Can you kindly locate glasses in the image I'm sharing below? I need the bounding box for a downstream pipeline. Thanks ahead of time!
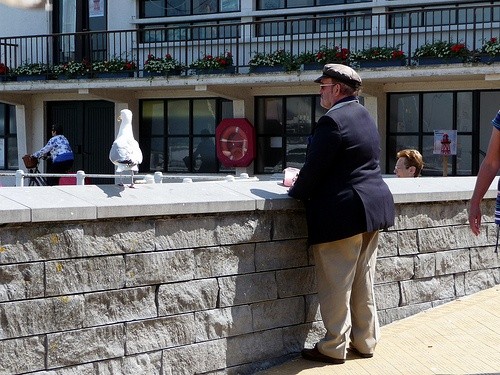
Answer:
[319,83,334,91]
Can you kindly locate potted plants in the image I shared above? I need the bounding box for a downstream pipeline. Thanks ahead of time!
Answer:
[59,168,91,184]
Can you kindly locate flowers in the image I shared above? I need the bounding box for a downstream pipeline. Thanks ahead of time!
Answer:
[0,38,500,76]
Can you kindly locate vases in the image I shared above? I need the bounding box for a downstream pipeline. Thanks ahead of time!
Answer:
[14,76,46,81]
[304,65,324,71]
[249,67,286,73]
[360,60,406,68]
[0,76,7,82]
[96,73,134,78]
[196,69,235,74]
[58,74,85,80]
[419,58,467,65]
[143,71,181,77]
[474,55,500,64]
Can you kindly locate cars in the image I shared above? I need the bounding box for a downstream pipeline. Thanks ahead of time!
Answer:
[272,153,306,173]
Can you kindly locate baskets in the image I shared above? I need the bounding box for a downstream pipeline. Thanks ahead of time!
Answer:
[23,156,37,168]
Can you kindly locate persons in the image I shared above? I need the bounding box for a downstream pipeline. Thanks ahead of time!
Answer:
[469,110,500,253]
[393,148,424,177]
[289,64,395,364]
[31,123,75,186]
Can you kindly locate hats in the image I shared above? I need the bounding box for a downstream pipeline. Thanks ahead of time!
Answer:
[314,63,362,90]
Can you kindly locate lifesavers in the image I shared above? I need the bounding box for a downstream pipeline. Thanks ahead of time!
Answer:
[220,126,248,161]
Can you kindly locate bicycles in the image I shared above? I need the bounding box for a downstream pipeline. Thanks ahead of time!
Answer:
[22,153,91,186]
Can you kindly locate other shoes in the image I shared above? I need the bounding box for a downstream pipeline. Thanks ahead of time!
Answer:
[349,342,373,358]
[300,343,344,363]
